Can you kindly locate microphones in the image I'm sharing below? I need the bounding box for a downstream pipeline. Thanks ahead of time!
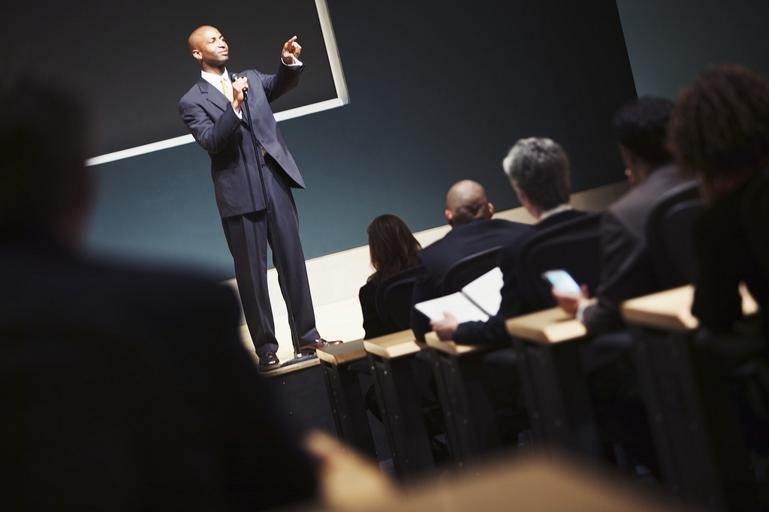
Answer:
[231,73,248,95]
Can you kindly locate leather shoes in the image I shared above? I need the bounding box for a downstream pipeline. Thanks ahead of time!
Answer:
[293,337,343,358]
[259,352,280,371]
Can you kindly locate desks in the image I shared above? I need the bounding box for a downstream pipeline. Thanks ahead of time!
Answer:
[317,284,760,483]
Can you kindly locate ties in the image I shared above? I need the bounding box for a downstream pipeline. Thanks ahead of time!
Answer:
[219,77,232,100]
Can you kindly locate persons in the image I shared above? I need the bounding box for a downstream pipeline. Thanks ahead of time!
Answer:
[176,25,344,373]
[2,71,322,511]
